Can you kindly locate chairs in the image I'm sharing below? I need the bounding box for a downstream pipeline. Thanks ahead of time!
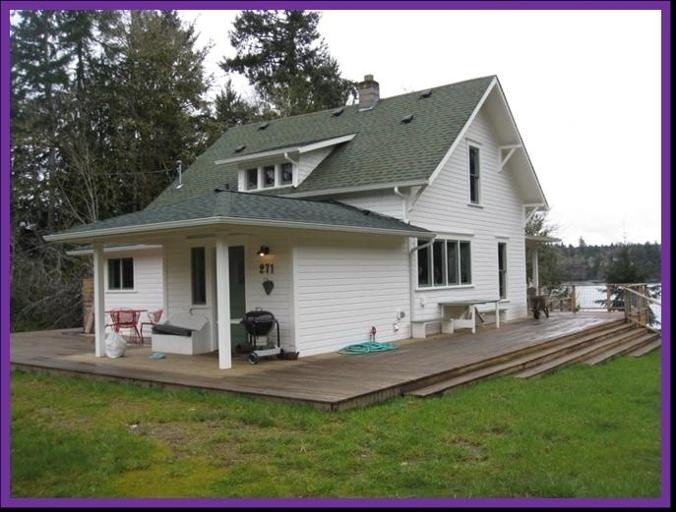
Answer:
[103,306,162,345]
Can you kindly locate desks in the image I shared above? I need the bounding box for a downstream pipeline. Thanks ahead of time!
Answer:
[436,296,500,334]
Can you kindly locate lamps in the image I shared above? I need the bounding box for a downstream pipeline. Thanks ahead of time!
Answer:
[256,245,269,256]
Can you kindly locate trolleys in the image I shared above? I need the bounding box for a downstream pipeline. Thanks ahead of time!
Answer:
[531,294,550,319]
[236,309,286,364]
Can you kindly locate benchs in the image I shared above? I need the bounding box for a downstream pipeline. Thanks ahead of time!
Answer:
[478,306,508,326]
[410,317,454,339]
[150,311,212,355]
